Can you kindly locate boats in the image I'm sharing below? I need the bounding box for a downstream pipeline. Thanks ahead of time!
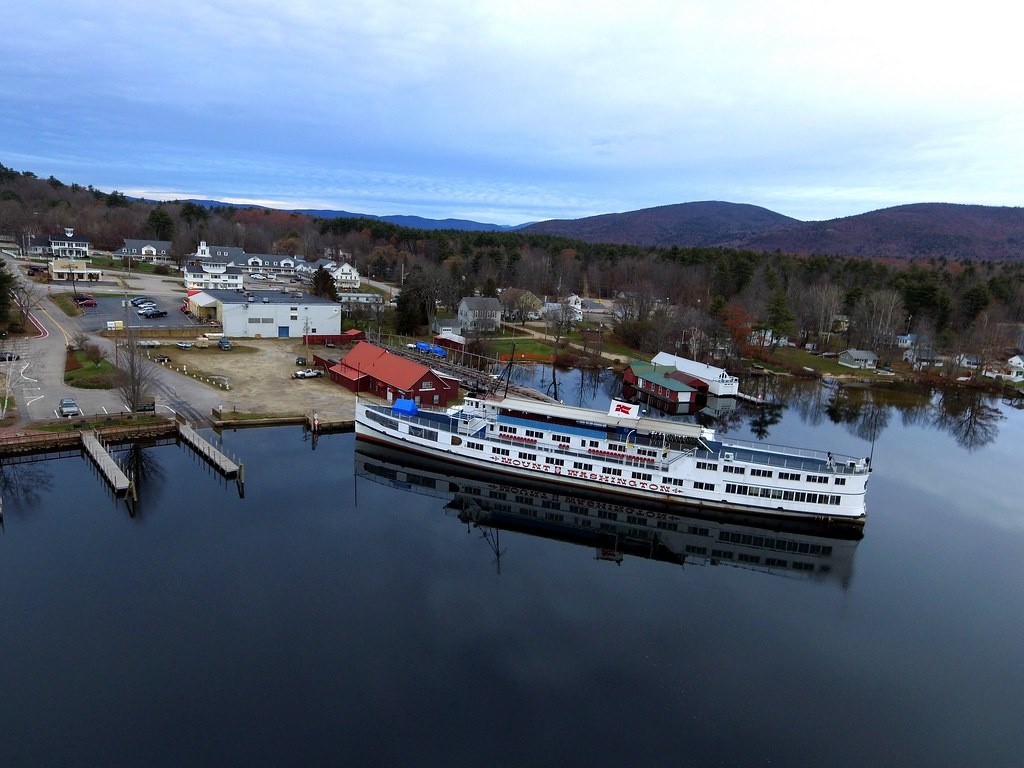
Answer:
[175,342,192,351]
[351,446,867,582]
[355,337,878,524]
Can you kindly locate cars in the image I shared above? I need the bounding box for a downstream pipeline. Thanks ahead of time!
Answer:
[71,292,98,307]
[59,398,79,417]
[295,357,307,367]
[0,351,20,362]
[130,296,168,319]
[242,291,269,304]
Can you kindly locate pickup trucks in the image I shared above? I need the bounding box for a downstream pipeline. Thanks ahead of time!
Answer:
[294,368,325,380]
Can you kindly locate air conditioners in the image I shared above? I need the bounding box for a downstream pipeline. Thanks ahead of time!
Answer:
[724,452,734,460]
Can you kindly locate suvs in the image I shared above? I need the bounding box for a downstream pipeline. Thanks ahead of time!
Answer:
[218,339,231,351]
